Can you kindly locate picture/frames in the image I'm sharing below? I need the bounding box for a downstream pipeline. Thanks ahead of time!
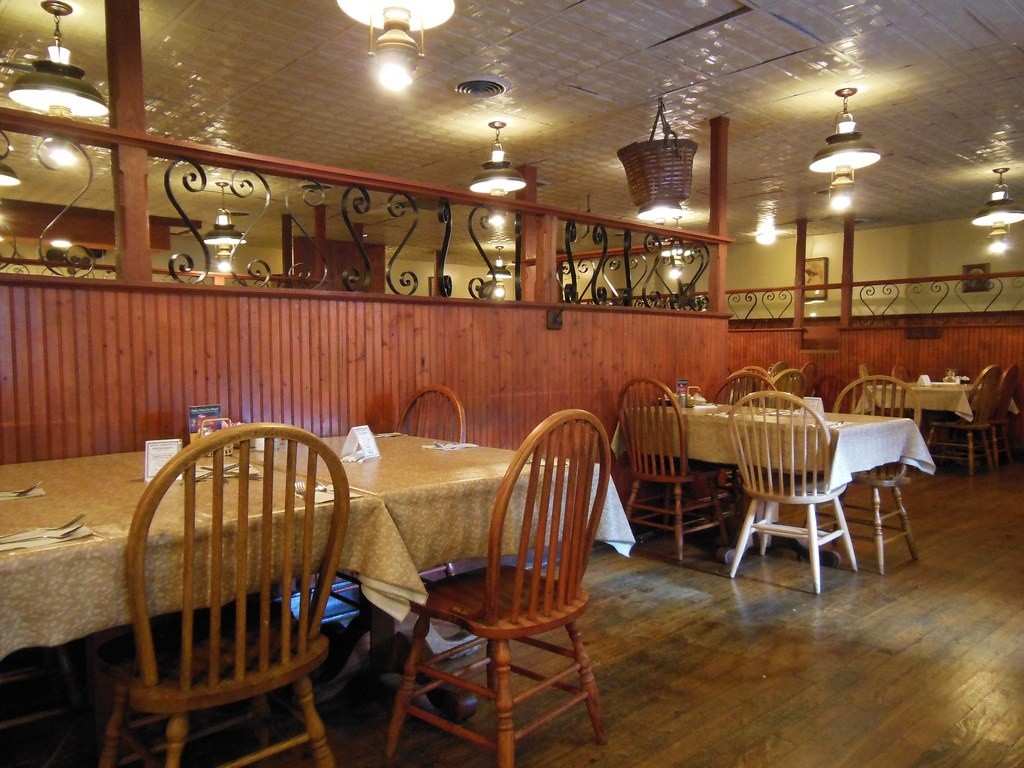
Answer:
[962,263,991,292]
[804,257,828,300]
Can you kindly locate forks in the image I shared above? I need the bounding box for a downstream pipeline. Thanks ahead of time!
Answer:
[12,481,40,493]
[0,513,85,539]
[294,482,306,495]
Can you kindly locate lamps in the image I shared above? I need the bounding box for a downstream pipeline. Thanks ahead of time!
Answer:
[618,96,700,223]
[486,246,512,296]
[0,143,22,188]
[204,180,247,272]
[809,89,881,213]
[9,0,109,171]
[971,167,1024,254]
[660,217,693,278]
[753,227,778,246]
[335,0,455,91]
[470,121,528,225]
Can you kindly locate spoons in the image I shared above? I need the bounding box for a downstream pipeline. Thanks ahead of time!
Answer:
[828,421,844,427]
[434,440,447,448]
[0,523,84,545]
[0,486,37,497]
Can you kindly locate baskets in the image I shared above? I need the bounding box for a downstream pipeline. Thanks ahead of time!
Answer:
[617,139,698,221]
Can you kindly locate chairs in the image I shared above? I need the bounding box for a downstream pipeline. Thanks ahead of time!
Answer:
[616,375,730,563]
[858,362,1019,475]
[727,390,857,594]
[713,373,779,550]
[729,359,818,397]
[327,385,466,636]
[91,422,349,768]
[385,409,610,768]
[796,376,919,576]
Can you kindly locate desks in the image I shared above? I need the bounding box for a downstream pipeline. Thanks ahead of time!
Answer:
[611,400,936,562]
[866,380,1019,422]
[0,432,636,728]
